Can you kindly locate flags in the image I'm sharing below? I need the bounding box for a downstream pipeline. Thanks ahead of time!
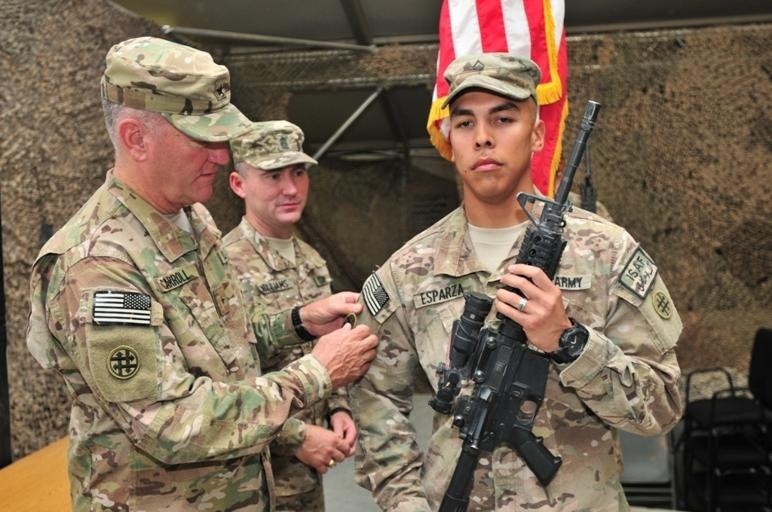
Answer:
[425,1,570,203]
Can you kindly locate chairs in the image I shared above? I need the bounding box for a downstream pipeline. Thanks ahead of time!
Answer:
[677,326,771,511]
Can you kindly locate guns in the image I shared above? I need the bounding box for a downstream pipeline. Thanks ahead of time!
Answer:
[428,100,602,512]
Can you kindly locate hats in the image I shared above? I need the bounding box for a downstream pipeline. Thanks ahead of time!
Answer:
[99,36,254,146]
[441,53,541,110]
[227,119,318,172]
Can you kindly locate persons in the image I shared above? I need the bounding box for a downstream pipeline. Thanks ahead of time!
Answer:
[349,52,685,512]
[219,119,358,511]
[27,35,381,512]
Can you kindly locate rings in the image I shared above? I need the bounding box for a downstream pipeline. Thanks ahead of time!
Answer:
[518,298,528,312]
[329,458,337,468]
